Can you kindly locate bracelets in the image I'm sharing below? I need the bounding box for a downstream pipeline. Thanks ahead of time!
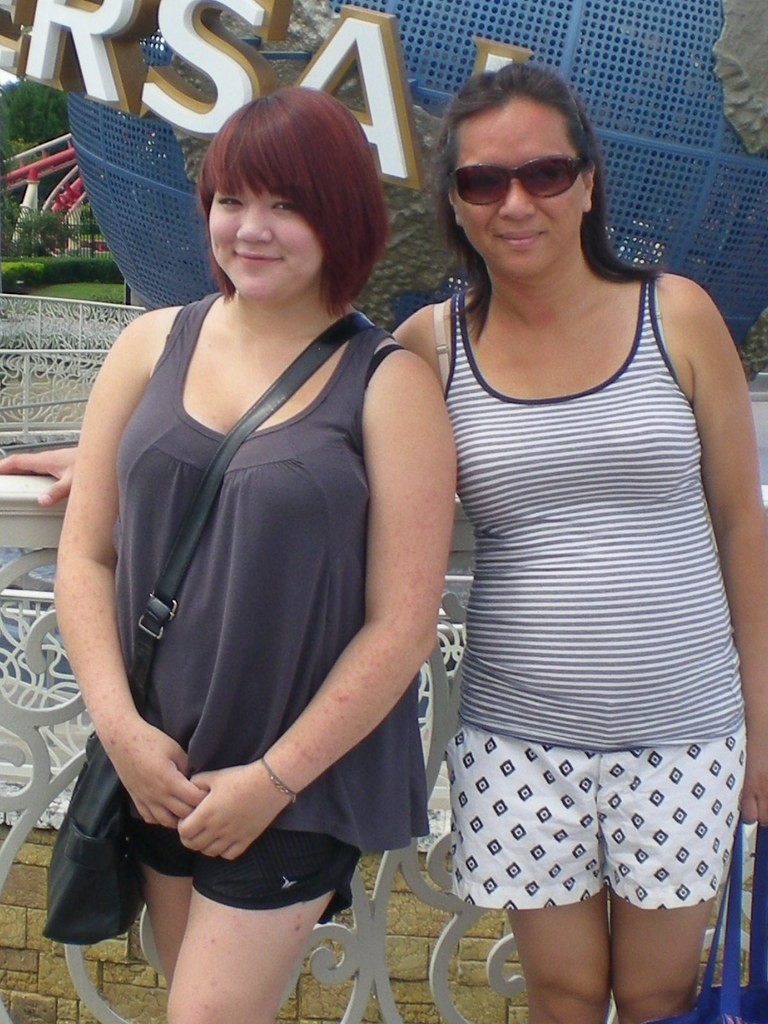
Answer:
[261,757,297,803]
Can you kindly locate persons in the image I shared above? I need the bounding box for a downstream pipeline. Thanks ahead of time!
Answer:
[0,64,767,1023]
[52,85,459,1023]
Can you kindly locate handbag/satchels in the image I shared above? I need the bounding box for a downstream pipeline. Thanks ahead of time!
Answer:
[643,811,768,1024]
[41,730,149,947]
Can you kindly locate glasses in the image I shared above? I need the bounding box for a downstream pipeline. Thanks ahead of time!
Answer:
[448,154,588,207]
